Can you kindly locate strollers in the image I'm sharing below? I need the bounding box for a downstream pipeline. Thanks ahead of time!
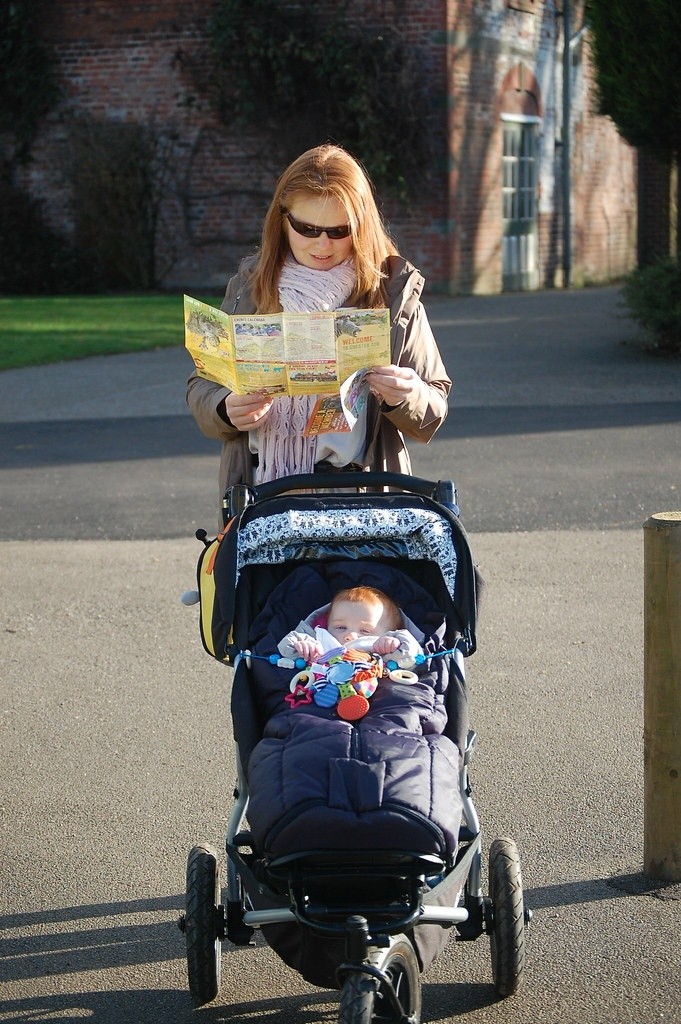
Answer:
[177,469,532,1024]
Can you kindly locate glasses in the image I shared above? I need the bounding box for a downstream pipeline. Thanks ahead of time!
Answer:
[283,208,351,239]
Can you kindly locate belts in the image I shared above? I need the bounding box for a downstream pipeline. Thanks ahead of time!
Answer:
[250,453,363,473]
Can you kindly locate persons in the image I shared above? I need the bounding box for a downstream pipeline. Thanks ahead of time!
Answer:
[186,144,453,532]
[247,586,465,895]
